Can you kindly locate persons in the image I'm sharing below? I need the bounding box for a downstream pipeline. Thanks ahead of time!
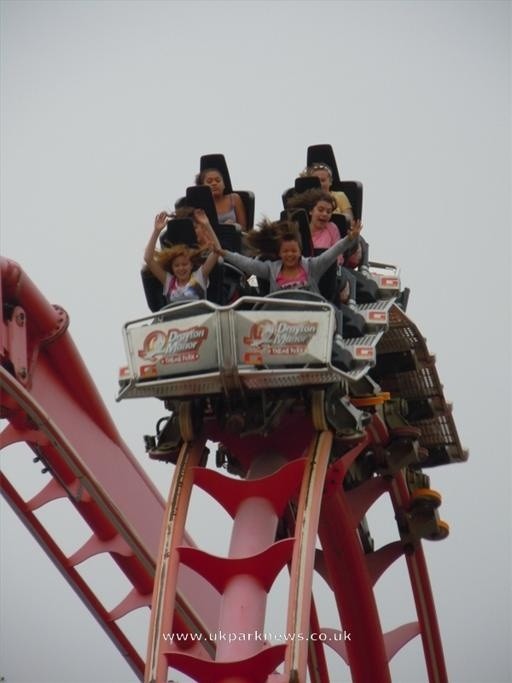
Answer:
[296,163,357,224]
[141,206,223,307]
[282,190,352,304]
[209,213,364,304]
[194,167,249,233]
[165,198,224,263]
